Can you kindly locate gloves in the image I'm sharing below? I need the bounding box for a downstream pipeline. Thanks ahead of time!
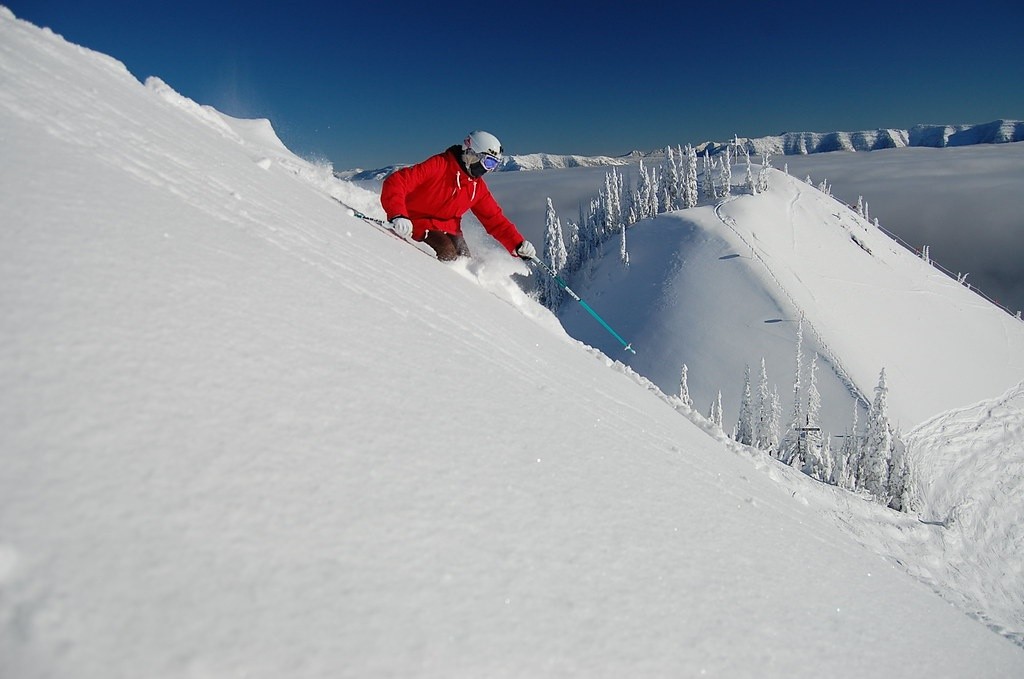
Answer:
[518,240,536,258]
[392,218,413,238]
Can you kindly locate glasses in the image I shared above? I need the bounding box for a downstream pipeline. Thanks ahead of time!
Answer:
[477,153,500,171]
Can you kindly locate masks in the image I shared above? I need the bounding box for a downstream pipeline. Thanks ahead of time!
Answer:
[469,161,488,177]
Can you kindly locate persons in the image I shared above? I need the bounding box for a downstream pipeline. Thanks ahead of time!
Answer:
[379,128,537,263]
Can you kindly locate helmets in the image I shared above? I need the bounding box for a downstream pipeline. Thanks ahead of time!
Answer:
[462,130,504,159]
